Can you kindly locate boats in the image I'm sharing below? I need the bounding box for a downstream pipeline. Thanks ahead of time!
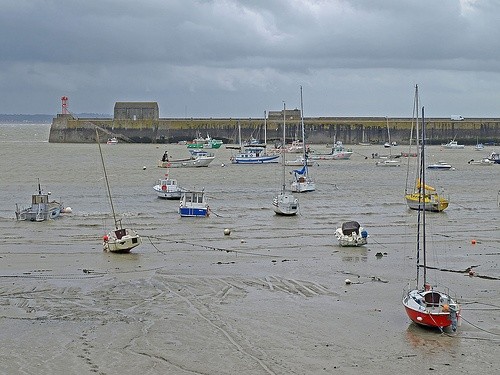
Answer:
[426,161,451,170]
[158,100,420,168]
[333,220,370,247]
[474,136,486,151]
[177,186,210,218]
[467,151,500,165]
[152,169,186,200]
[14,178,72,223]
[441,132,465,149]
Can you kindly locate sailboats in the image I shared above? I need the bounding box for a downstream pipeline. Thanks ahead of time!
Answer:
[404,83,449,211]
[272,100,299,216]
[402,106,462,333]
[288,84,316,194]
[95,128,143,255]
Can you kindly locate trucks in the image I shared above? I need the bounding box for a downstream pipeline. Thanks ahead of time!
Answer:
[450,115,464,121]
